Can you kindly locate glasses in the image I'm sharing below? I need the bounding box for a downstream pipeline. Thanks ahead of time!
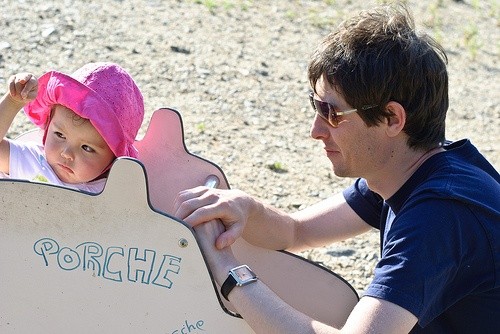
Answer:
[309,93,379,127]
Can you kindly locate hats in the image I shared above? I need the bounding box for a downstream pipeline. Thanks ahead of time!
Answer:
[24,63,145,158]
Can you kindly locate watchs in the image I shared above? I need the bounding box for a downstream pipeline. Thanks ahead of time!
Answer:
[220,264,260,302]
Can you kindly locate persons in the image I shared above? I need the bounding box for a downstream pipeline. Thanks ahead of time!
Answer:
[168,0,500,334]
[0,66,141,198]
